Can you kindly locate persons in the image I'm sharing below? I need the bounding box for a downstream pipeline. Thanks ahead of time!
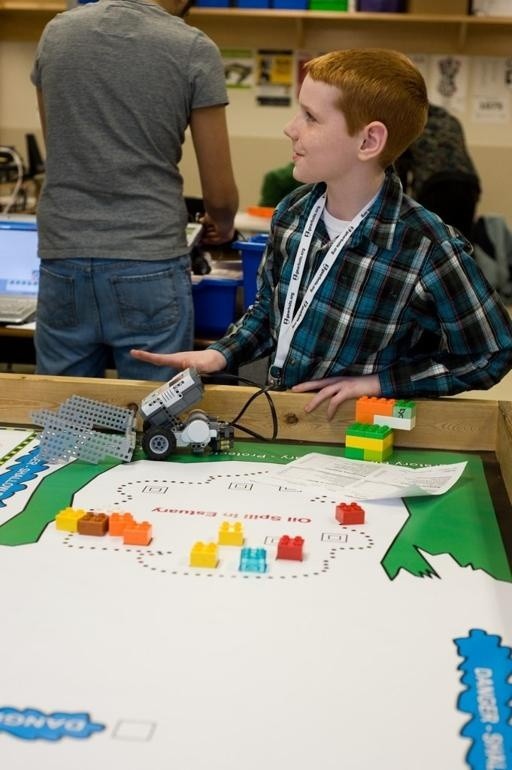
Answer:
[30,1,240,383]
[130,47,511,422]
[395,106,511,307]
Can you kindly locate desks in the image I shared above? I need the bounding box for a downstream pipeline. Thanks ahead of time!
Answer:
[0,373,512,770]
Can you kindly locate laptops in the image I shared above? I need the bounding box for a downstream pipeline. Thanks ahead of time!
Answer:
[0,213,42,325]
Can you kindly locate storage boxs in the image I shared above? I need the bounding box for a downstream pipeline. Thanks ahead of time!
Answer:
[233,234,269,313]
[196,279,243,338]
